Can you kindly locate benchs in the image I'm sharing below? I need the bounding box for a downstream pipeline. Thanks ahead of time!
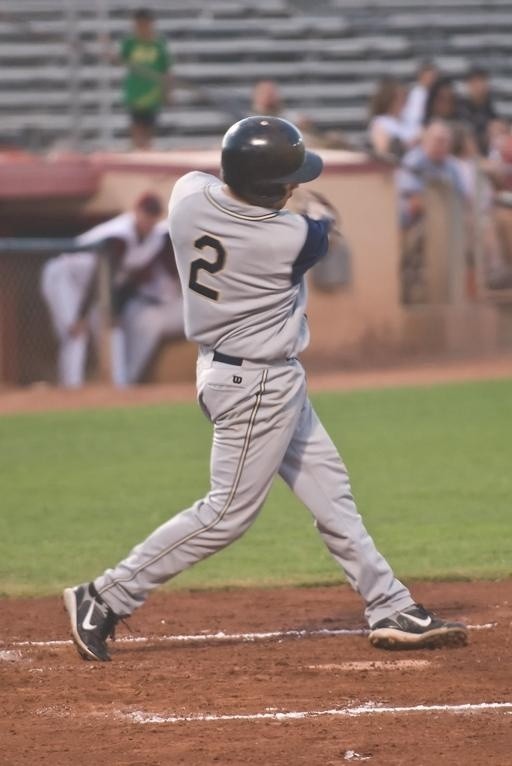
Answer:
[1,1,512,150]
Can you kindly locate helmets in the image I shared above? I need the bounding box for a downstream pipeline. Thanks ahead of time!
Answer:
[220,115,324,184]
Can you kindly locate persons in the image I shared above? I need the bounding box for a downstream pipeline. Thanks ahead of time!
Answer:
[115,237,186,386]
[57,111,471,667]
[242,80,325,150]
[366,55,512,291]
[107,8,178,152]
[40,192,170,385]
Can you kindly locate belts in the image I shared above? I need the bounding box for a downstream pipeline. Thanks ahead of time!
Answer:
[212,351,243,366]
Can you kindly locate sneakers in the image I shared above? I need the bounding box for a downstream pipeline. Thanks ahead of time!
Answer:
[368,602,469,650]
[63,582,115,662]
